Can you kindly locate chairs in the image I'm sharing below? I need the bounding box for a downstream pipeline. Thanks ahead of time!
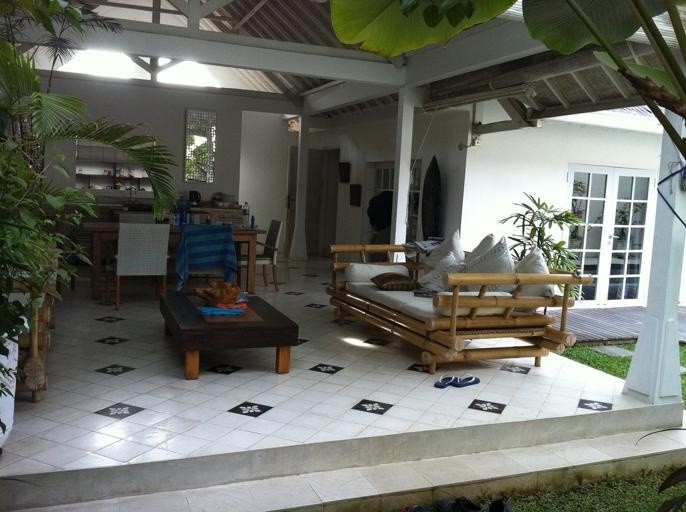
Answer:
[327,240,594,373]
[113,209,245,309]
[235,220,284,292]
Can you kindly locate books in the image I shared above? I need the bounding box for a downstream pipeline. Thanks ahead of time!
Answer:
[414,240,441,254]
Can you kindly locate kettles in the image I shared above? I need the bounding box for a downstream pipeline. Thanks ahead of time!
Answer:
[189,190,202,205]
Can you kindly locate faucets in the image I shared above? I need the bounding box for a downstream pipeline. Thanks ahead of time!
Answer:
[128,185,136,202]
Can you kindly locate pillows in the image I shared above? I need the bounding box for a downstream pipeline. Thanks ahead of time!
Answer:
[416,228,552,299]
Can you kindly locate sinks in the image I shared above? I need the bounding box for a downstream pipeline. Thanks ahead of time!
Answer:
[122,203,152,211]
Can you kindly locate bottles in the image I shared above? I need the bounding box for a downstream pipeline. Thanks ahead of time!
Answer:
[241,201,250,227]
[252,215,256,229]
